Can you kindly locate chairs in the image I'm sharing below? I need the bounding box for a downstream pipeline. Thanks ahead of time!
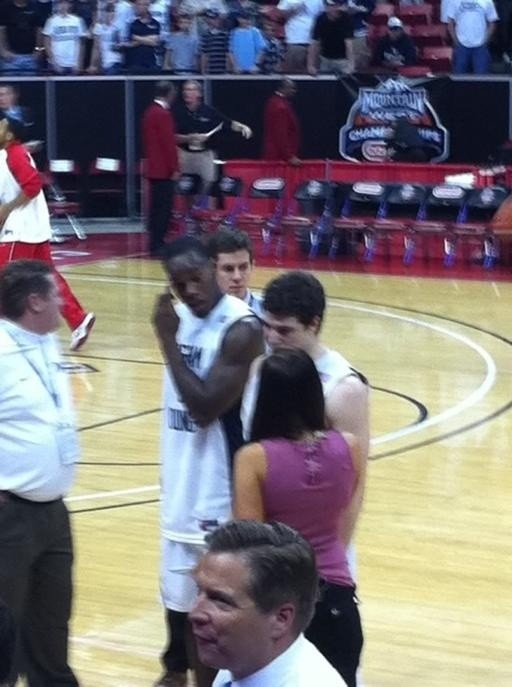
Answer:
[252,0,455,79]
[38,171,87,242]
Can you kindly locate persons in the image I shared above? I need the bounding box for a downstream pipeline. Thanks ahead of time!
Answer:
[239,271,370,588]
[0,259,80,687]
[144,80,180,259]
[234,348,367,687]
[173,78,252,153]
[0,0,512,78]
[385,116,429,165]
[187,522,347,687]
[260,79,302,165]
[1,83,96,351]
[487,138,511,166]
[154,237,266,687]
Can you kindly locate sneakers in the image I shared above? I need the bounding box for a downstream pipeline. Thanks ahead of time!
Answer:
[152,670,188,687]
[68,311,96,351]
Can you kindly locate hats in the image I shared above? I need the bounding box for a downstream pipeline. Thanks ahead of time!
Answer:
[387,17,405,30]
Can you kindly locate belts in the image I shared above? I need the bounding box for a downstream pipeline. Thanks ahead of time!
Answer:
[1,490,25,503]
